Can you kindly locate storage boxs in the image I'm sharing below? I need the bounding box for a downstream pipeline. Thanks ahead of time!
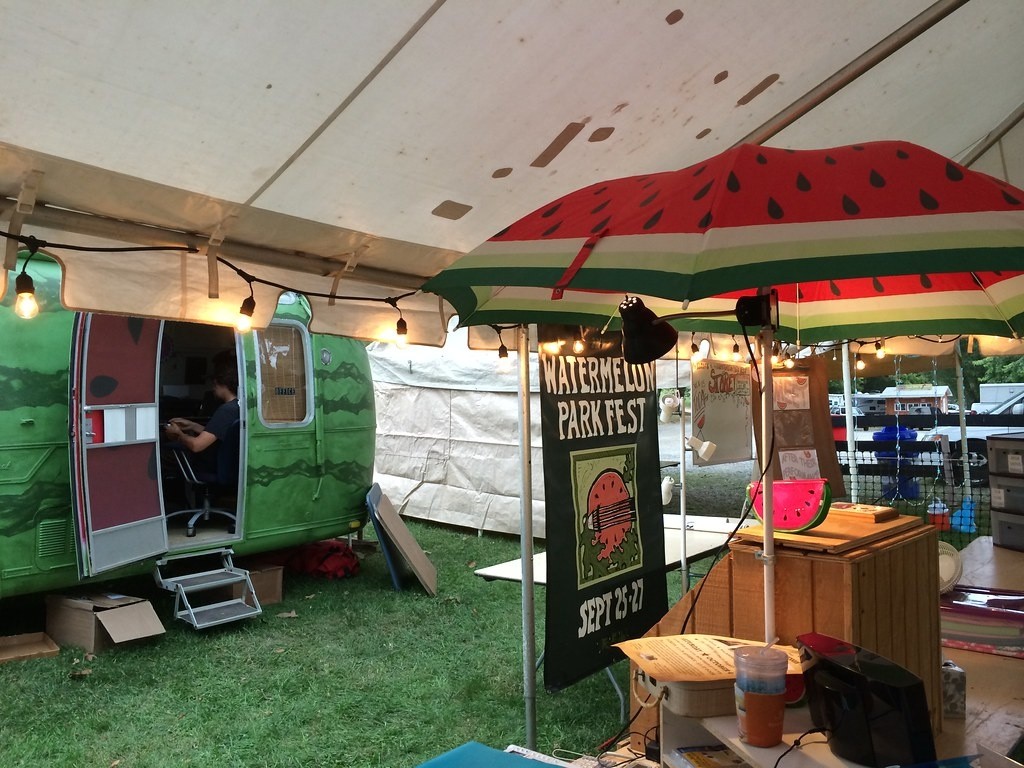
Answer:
[0,632,60,664]
[45,591,166,654]
[986,432,1024,552]
[222,562,284,605]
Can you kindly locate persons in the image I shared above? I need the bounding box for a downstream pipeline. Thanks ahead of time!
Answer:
[165,375,239,525]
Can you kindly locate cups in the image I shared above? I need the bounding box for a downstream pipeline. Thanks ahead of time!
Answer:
[734,646,789,694]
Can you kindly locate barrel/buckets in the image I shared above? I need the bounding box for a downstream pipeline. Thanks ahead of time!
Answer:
[926,497,950,531]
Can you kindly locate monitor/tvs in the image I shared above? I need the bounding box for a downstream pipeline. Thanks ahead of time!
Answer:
[795,631,939,768]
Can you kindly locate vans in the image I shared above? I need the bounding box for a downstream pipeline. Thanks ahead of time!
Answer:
[0,249,378,632]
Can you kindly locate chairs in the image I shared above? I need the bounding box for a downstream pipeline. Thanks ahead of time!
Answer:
[164,441,236,538]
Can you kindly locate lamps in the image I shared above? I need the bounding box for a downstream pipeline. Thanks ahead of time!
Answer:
[661,477,675,506]
[618,294,768,365]
[659,396,679,424]
[685,436,717,461]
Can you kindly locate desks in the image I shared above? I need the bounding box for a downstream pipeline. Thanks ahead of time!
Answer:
[474,514,761,725]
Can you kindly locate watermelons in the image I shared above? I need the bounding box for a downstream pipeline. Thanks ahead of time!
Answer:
[746,479,831,534]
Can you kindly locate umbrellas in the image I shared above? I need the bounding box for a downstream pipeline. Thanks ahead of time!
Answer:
[782,332,1024,379]
[414,141,1023,637]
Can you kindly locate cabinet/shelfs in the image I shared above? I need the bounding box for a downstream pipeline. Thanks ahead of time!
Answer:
[660,701,900,768]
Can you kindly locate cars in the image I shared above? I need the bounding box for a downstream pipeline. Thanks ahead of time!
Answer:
[916,391,1024,488]
[831,406,869,431]
[908,406,943,430]
[948,404,977,415]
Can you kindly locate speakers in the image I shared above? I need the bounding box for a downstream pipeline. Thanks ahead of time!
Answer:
[736,295,771,326]
